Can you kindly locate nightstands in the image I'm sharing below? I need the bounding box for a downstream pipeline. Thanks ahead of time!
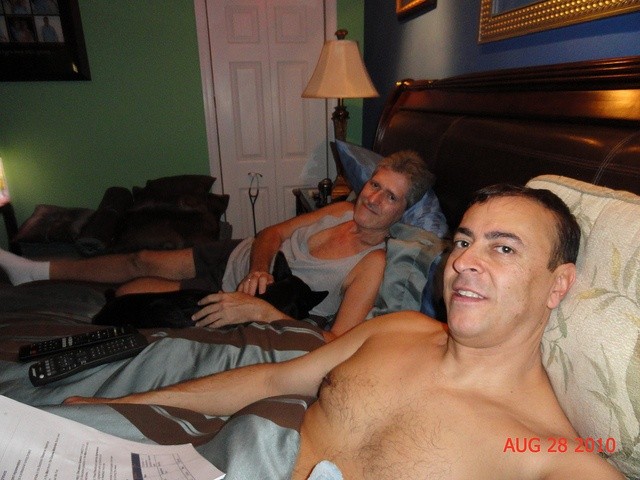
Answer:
[292,187,348,217]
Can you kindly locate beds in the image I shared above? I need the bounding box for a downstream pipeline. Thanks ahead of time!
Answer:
[1,58,640,480]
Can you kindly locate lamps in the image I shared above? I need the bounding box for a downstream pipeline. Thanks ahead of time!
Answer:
[301,30,379,198]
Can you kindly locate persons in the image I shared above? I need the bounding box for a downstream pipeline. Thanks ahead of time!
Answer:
[0,150,427,344]
[62,183,628,480]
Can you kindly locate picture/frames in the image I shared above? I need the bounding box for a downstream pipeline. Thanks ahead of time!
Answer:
[477,0,640,45]
[1,0,95,82]
[394,0,425,15]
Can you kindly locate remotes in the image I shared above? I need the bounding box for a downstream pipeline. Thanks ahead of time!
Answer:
[30,333,149,385]
[19,324,139,363]
